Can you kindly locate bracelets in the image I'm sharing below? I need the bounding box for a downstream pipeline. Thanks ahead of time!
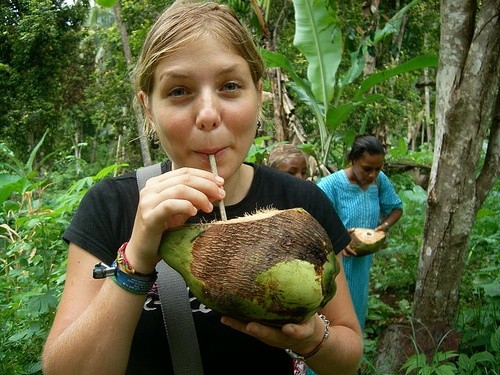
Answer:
[285,314,330,360]
[384,221,390,229]
[93,241,158,295]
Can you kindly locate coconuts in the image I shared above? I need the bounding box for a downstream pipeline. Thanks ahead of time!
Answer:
[158,206,341,328]
[348,228,387,256]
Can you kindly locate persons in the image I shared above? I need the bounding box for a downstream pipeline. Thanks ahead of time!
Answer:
[267,143,308,374]
[40,1,364,375]
[303,134,403,374]
